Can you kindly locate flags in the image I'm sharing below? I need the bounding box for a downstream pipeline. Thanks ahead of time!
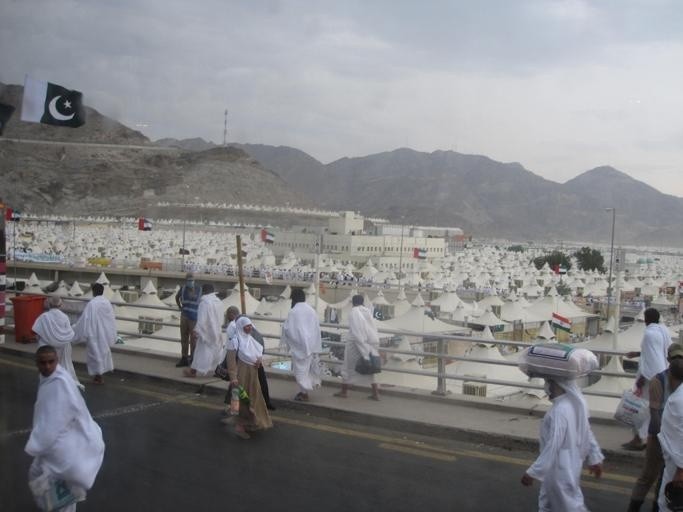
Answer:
[413,247,426,259]
[138,218,152,231]
[20,73,86,129]
[260,229,274,244]
[4,207,21,222]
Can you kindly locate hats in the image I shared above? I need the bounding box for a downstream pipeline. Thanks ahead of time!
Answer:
[668,343,683,358]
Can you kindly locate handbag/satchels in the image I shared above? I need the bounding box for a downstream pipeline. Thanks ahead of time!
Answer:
[28,464,87,512]
[355,352,382,374]
[614,388,650,429]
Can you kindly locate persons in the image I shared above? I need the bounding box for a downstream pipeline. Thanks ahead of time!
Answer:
[174,271,382,441]
[70,283,117,385]
[24,344,104,512]
[32,295,81,391]
[520,308,683,512]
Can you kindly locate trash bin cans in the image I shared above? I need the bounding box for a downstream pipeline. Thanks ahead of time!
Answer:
[9,295,47,344]
